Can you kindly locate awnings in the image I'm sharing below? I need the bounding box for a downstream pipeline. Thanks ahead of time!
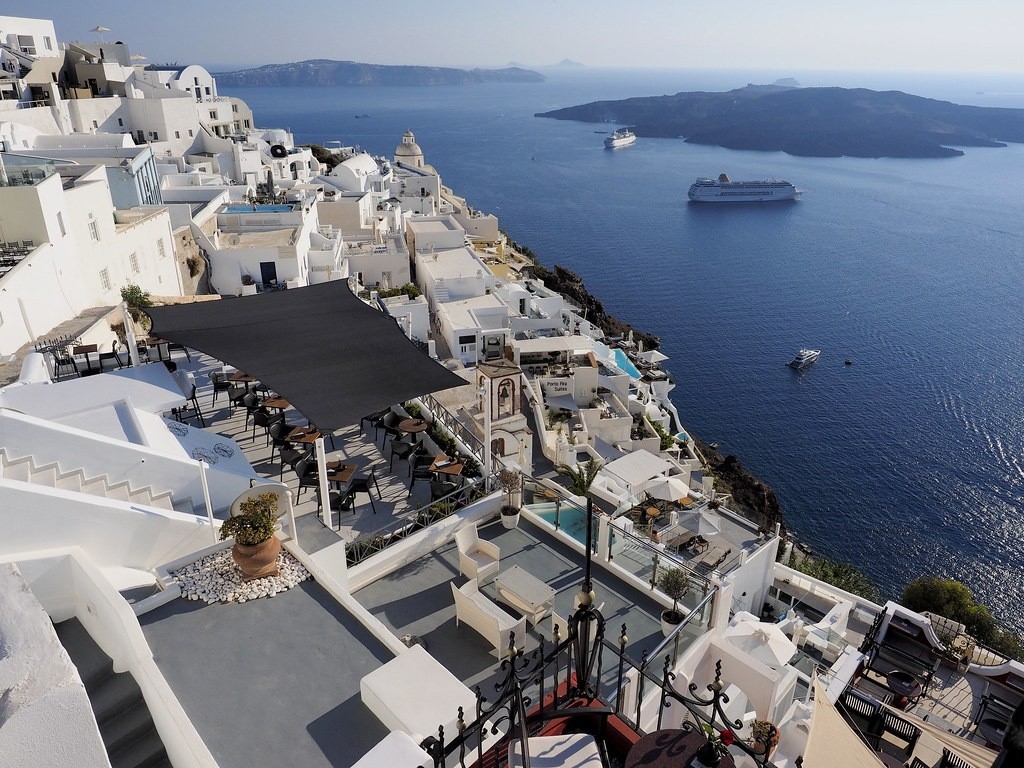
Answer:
[594,434,628,464]
[138,276,472,440]
[544,392,580,412]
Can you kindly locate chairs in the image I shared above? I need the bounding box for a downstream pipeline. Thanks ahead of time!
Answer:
[974,672,1024,725]
[98,340,124,373]
[452,523,502,583]
[407,453,439,499]
[175,384,206,429]
[167,343,193,363]
[294,459,323,505]
[429,476,465,503]
[348,464,382,514]
[903,754,930,768]
[314,481,355,530]
[838,690,881,734]
[853,727,880,751]
[52,353,80,380]
[880,711,922,757]
[550,603,605,650]
[505,681,613,768]
[126,341,151,367]
[208,370,235,408]
[228,368,469,482]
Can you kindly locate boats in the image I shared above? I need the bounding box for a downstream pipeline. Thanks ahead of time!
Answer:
[603,128,636,148]
[789,348,820,369]
[687,173,796,202]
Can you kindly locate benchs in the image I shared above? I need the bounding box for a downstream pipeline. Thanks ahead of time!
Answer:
[849,674,895,709]
[449,577,528,662]
[863,641,942,704]
[699,546,731,571]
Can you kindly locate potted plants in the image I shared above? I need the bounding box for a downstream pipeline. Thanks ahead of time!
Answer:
[218,491,283,582]
[495,470,522,530]
[658,566,689,640]
[749,718,781,756]
[763,602,773,619]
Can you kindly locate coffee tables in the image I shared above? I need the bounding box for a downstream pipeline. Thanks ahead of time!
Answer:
[493,565,558,628]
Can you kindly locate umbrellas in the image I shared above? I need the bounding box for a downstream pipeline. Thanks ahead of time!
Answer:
[644,476,690,518]
[130,54,146,64]
[638,349,670,371]
[727,619,797,668]
[89,25,112,43]
[677,509,720,554]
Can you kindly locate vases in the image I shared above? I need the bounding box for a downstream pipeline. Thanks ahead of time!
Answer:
[697,742,722,766]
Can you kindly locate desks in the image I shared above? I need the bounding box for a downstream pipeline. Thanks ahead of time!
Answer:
[316,461,358,489]
[262,392,290,413]
[430,454,468,481]
[284,427,320,450]
[398,419,428,443]
[229,370,255,393]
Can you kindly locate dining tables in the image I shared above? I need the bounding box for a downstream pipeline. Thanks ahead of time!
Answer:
[72,344,99,377]
[146,338,177,373]
[624,729,735,768]
[884,672,924,711]
[695,541,709,553]
[979,718,1007,752]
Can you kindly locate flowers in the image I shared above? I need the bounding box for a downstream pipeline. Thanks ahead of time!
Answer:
[702,723,733,746]
